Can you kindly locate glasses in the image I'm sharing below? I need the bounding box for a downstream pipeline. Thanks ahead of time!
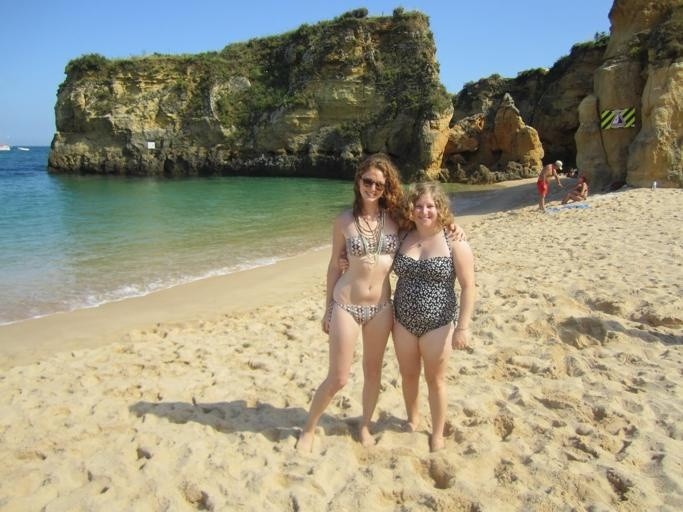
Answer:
[360,177,386,191]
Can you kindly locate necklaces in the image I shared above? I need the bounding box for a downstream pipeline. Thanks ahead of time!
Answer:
[416,232,429,246]
[353,208,385,271]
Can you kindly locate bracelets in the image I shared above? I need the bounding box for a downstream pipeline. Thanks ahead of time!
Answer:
[456,325,469,331]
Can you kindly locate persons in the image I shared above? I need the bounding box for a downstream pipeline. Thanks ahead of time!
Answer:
[562,176,588,204]
[339,181,474,453]
[537,160,563,210]
[295,154,466,453]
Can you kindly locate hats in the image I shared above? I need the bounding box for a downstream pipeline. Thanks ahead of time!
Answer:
[555,160,563,170]
